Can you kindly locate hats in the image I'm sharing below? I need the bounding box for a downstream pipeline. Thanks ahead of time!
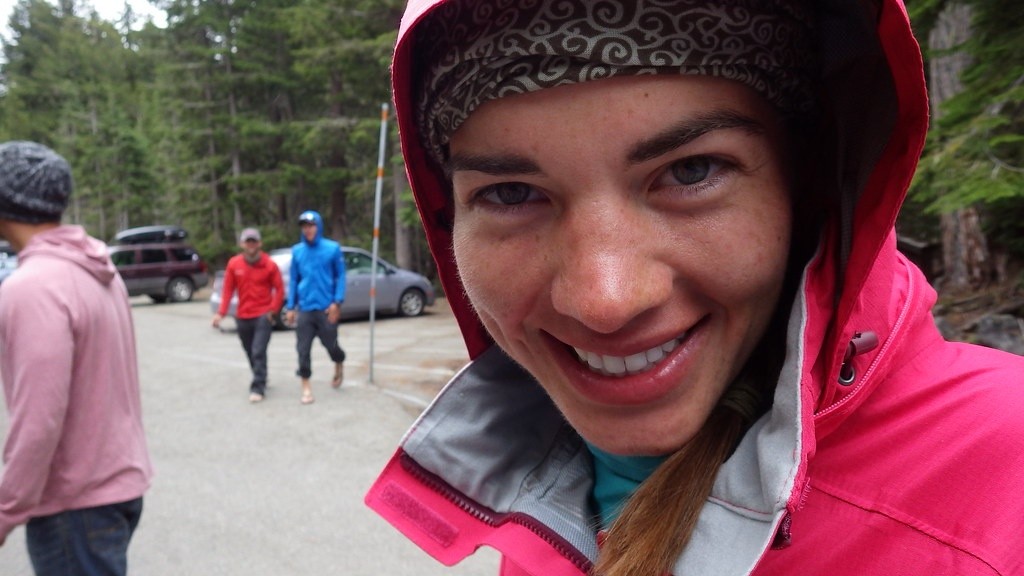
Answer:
[0,140,73,224]
[297,210,324,223]
[240,227,263,243]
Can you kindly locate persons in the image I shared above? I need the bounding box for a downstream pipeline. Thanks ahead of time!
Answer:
[210,229,284,403]
[0,142,155,575]
[365,1,1024,576]
[285,210,347,404]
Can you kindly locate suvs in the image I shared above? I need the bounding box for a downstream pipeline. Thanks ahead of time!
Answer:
[108,226,209,305]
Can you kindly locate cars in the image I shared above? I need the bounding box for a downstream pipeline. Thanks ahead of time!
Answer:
[210,240,434,327]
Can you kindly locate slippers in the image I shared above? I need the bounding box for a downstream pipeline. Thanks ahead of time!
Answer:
[300,389,316,406]
[249,392,265,405]
[331,368,343,388]
